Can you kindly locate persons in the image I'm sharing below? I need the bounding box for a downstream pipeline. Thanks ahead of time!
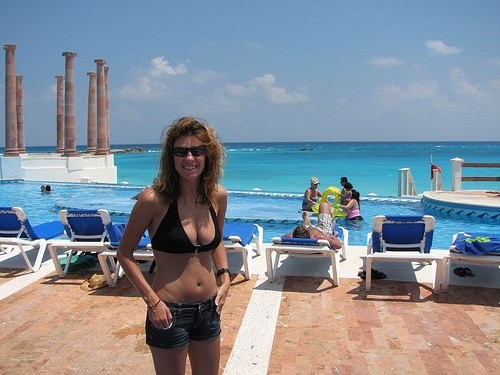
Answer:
[40,184,51,192]
[115,118,231,375]
[280,175,364,250]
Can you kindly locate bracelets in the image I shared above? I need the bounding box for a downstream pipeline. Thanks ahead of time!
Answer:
[217,268,231,279]
[147,299,161,310]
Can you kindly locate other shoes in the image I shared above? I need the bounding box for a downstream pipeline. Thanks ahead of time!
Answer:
[454,267,475,277]
[358,268,386,279]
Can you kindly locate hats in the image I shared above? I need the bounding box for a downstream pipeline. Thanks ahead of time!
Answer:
[311,177,320,184]
[81,272,111,291]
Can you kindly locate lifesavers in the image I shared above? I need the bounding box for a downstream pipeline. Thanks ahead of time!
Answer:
[311,204,346,217]
[321,187,341,207]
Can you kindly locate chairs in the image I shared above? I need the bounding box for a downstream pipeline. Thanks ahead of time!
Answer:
[217,222,263,281]
[0,204,64,273]
[360,215,500,292]
[265,226,349,286]
[47,209,155,288]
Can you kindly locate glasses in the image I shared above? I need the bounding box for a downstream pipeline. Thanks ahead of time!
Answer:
[172,147,205,157]
